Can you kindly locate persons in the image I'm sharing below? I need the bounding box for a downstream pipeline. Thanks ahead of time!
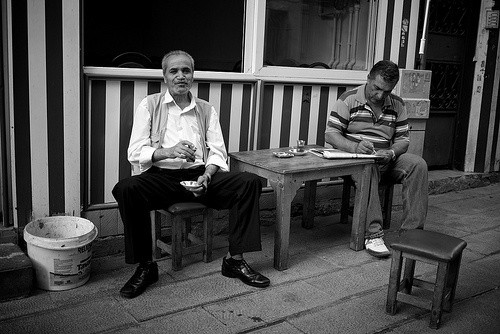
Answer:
[112,50,270,298]
[325,60,428,257]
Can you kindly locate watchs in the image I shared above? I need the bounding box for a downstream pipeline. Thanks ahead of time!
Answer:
[204,173,211,185]
[389,149,396,160]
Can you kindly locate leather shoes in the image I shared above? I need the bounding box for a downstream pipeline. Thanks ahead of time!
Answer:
[222,256,270,287]
[119,261,159,298]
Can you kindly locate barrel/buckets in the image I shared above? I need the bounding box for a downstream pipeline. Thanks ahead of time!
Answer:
[23,216,99,292]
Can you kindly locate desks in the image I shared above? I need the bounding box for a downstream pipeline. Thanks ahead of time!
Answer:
[228,145,375,271]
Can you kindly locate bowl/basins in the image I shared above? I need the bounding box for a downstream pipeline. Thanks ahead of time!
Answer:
[180,181,202,190]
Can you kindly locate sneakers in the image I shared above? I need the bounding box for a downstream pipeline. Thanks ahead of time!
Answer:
[364,237,390,257]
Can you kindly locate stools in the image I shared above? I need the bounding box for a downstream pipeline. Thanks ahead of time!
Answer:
[151,202,213,274]
[385,228,468,330]
[342,176,395,230]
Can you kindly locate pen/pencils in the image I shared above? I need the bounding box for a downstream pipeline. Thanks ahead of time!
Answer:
[360,136,377,154]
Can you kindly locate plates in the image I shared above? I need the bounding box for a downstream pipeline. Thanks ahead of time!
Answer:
[272,152,294,157]
[289,149,308,156]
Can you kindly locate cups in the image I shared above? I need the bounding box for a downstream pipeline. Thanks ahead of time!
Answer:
[297,140,305,152]
